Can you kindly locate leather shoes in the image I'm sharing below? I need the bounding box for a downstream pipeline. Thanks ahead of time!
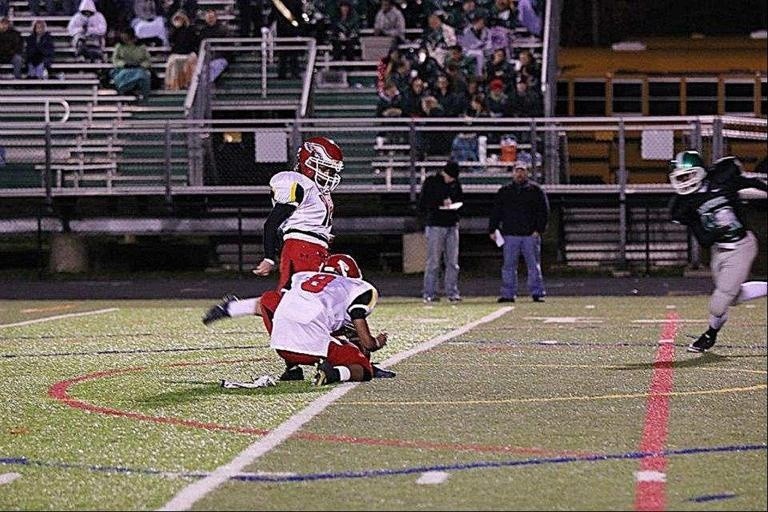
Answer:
[497,297,515,302]
[532,294,545,302]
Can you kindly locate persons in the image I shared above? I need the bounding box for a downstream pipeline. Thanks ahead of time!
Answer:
[489,160,549,303]
[24,20,55,80]
[266,0,303,81]
[269,253,387,386]
[236,0,263,53]
[202,138,344,376]
[134,1,168,60]
[46,1,74,17]
[157,0,176,33]
[332,0,360,88]
[300,1,333,44]
[199,9,235,91]
[67,0,107,64]
[418,160,464,303]
[361,0,547,168]
[666,151,767,351]
[0,18,24,79]
[112,27,153,104]
[0,1,41,18]
[164,10,198,92]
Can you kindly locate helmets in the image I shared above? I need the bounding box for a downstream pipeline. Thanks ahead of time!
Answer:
[297,135,345,195]
[668,150,706,194]
[318,252,363,279]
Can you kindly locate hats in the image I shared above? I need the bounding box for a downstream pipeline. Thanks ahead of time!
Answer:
[443,161,460,180]
[514,160,527,170]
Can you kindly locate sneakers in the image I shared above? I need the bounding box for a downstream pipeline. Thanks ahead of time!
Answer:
[313,357,339,386]
[202,293,242,326]
[447,294,461,302]
[424,295,440,302]
[278,365,304,381]
[692,330,716,349]
